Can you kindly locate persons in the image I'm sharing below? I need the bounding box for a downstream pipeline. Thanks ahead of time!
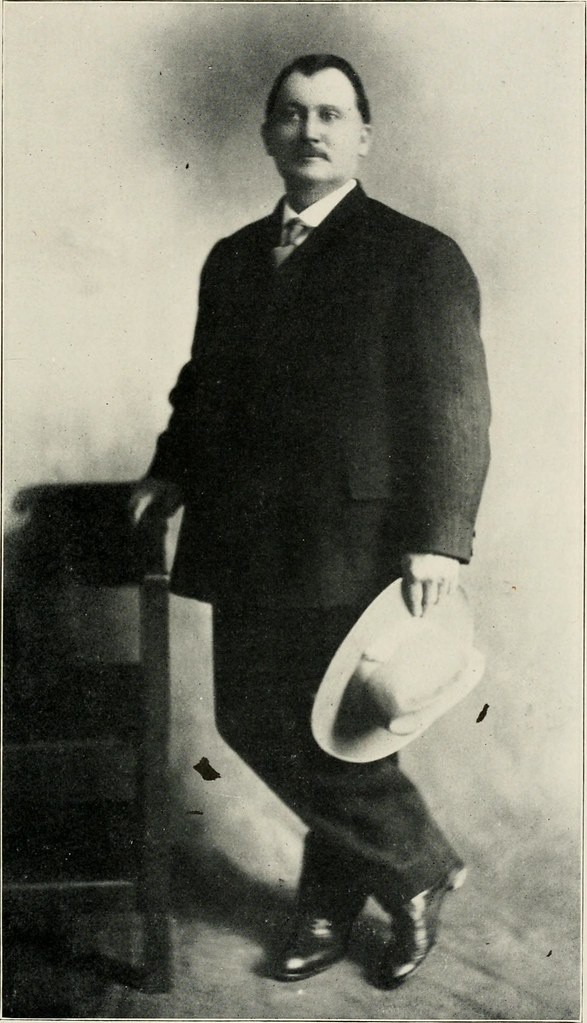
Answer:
[120,52,491,988]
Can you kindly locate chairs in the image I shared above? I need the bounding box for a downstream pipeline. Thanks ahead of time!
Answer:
[3,555,173,997]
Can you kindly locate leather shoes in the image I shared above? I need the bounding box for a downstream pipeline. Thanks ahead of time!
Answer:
[377,857,467,981]
[275,830,374,979]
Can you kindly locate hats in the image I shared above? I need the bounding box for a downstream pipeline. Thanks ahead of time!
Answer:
[310,577,485,762]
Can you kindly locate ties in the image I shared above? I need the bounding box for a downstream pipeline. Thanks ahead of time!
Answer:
[280,221,302,246]
